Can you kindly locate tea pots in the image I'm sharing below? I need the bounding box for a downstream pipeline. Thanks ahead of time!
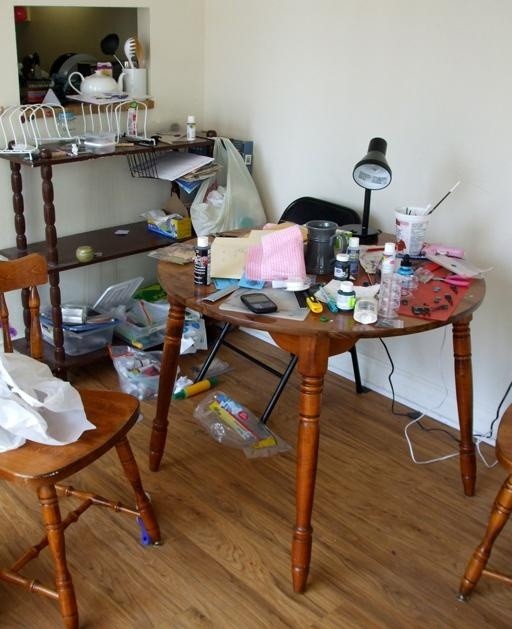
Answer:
[68,70,126,98]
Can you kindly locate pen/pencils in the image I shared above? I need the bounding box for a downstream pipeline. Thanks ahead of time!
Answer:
[318,284,338,313]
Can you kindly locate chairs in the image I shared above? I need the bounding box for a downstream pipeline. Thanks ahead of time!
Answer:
[0,254,162,628]
[459,403,511,603]
[193,197,362,424]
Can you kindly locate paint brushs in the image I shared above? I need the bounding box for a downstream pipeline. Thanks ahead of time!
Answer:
[427,182,461,216]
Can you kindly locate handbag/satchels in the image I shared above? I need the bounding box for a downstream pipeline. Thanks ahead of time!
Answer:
[0,352,97,452]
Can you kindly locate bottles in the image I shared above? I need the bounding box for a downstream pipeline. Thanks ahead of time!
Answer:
[382,241,396,274]
[193,235,211,286]
[126,101,138,138]
[346,237,360,273]
[186,116,196,141]
[51,111,80,148]
[335,253,349,279]
[338,281,356,311]
[398,254,414,297]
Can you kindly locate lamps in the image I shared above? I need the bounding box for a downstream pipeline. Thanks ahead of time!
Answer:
[340,138,391,245]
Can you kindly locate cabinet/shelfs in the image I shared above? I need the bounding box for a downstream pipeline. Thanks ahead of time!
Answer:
[1,130,216,382]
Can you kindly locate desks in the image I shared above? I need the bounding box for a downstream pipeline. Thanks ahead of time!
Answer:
[152,227,486,593]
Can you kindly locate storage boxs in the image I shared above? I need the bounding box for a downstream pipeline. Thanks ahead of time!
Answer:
[40,301,123,357]
[93,277,169,342]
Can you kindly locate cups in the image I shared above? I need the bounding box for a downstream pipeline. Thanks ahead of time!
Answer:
[378,273,402,317]
[353,297,378,325]
[395,206,430,257]
[119,69,146,100]
[306,221,338,275]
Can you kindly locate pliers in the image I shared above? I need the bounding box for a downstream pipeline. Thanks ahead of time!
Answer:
[432,273,473,287]
